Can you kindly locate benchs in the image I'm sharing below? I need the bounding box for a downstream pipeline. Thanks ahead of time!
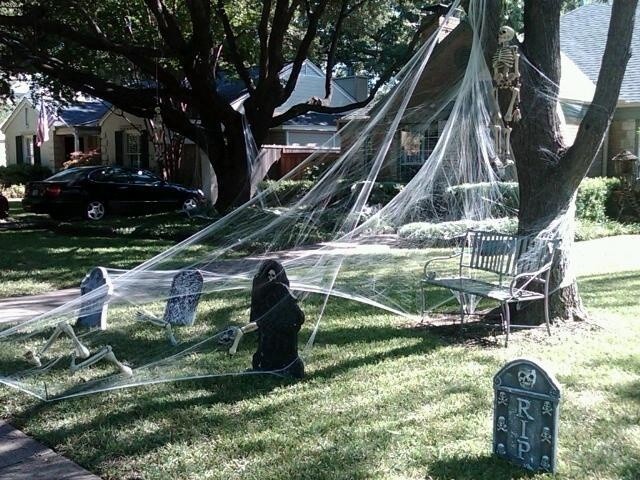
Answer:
[420,226,562,349]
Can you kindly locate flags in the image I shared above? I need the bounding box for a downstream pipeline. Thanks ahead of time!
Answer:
[34,92,56,147]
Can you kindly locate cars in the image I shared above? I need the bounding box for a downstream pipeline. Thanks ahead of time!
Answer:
[21,162,211,225]
